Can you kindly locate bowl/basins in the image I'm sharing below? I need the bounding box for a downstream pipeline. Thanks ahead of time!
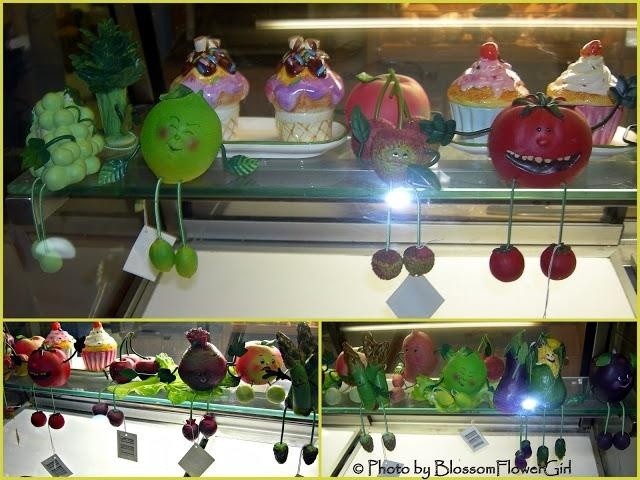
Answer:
[448,100,506,144]
[63,349,78,365]
[81,350,117,371]
[569,105,624,147]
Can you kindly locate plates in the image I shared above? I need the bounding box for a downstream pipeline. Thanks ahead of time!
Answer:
[443,124,639,162]
[65,356,120,378]
[214,115,349,159]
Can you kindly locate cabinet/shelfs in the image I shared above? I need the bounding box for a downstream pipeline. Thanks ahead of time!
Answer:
[6,139,636,430]
